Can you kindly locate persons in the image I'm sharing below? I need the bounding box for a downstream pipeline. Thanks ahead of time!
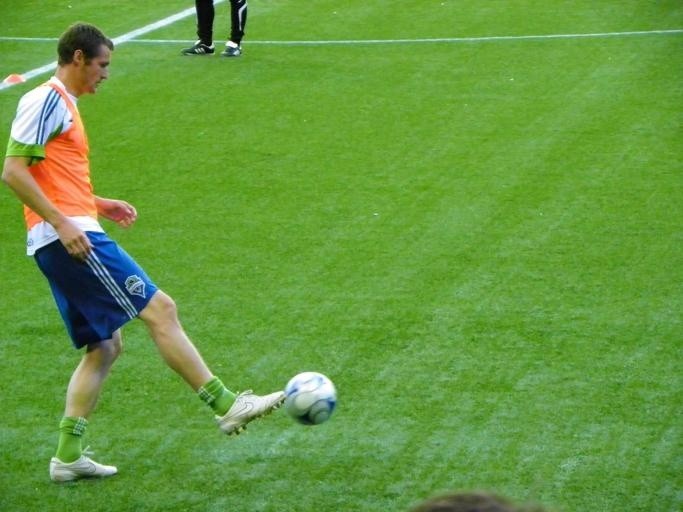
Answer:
[180,0,249,59]
[0,22,286,484]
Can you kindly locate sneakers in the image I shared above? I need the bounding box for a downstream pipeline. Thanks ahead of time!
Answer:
[48,445,117,483]
[220,41,241,56]
[213,389,287,436]
[180,39,214,56]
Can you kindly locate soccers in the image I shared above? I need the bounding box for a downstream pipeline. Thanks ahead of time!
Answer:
[284,370,338,427]
